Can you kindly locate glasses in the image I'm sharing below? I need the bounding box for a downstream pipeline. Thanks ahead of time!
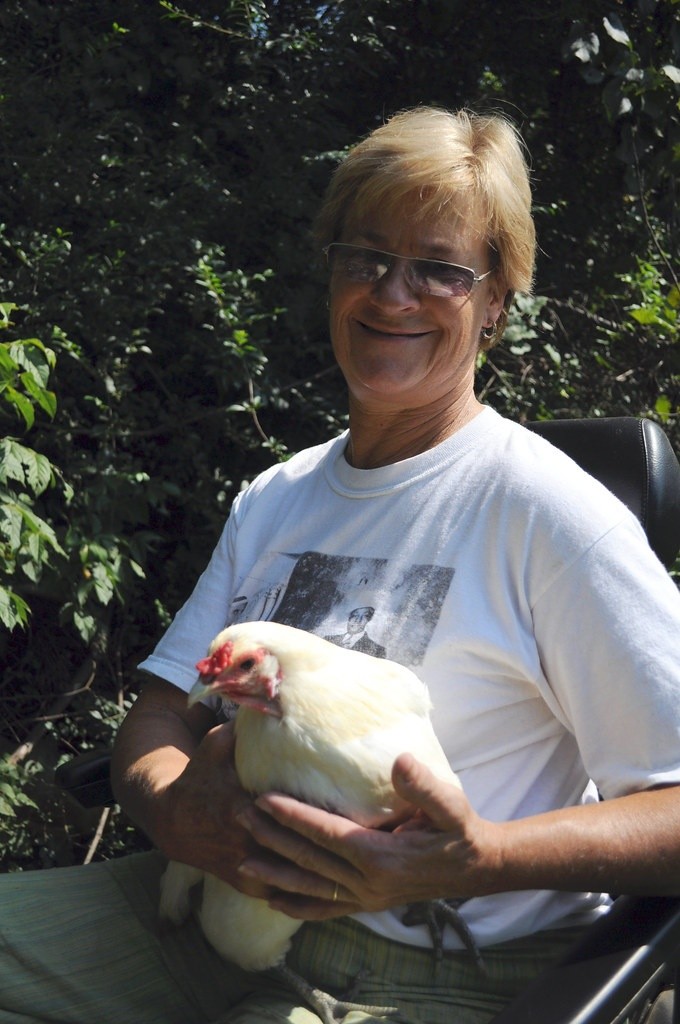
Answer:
[322,240,500,298]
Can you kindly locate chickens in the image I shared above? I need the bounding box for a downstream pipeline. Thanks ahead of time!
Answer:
[151,621,482,1024]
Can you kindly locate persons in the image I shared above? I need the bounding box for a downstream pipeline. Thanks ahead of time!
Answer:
[0,106,680,1024]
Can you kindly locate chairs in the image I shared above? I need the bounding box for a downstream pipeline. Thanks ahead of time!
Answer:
[492,416,680,1024]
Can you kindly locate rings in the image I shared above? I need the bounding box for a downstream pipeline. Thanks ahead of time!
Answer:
[332,882,340,903]
